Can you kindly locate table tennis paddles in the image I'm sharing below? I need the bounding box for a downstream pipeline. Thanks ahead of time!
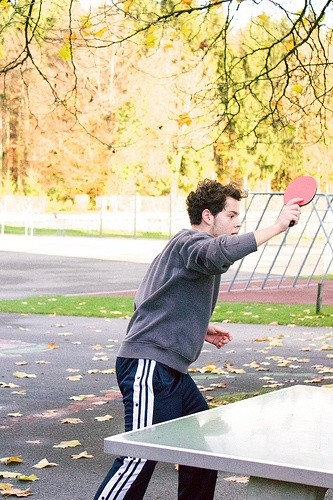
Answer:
[283,176,317,228]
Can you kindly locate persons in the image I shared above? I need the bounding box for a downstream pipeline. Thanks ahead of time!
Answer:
[95,179,304,500]
